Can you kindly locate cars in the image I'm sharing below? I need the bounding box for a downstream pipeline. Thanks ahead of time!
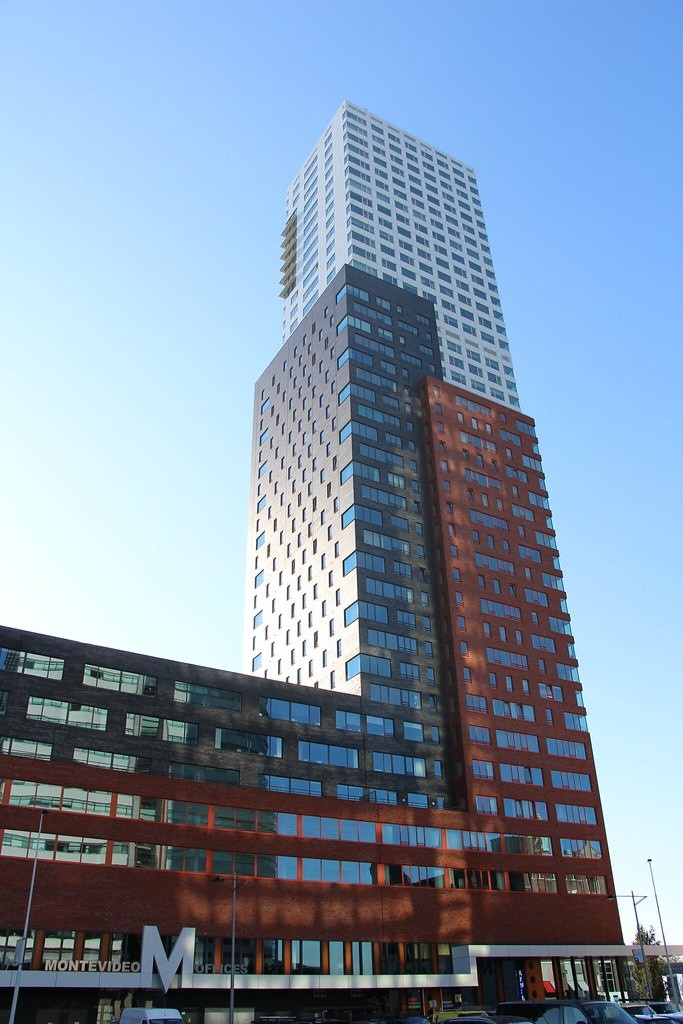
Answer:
[589,1002,683,1024]
[431,1016,536,1024]
[369,1012,431,1024]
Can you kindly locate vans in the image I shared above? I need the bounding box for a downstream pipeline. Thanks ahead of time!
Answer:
[496,1001,640,1024]
[119,1008,184,1024]
[432,1010,490,1022]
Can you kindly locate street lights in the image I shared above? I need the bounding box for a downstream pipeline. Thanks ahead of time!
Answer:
[216,876,237,1024]
[10,809,46,1024]
[630,859,656,1001]
[607,895,654,1001]
